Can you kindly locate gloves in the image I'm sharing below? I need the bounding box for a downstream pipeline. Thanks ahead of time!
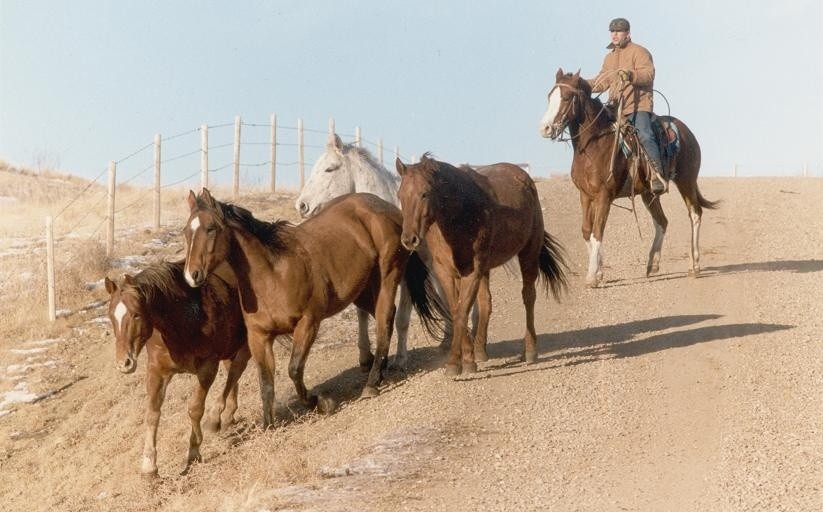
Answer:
[618,68,630,82]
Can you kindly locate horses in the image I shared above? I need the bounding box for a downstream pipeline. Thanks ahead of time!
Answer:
[295,128,479,368]
[395,157,568,377]
[537,65,722,290]
[179,187,452,434]
[103,250,293,480]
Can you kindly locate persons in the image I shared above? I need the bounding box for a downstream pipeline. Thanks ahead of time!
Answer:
[586,18,669,191]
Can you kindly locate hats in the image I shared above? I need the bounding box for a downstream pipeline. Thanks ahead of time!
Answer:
[608,18,630,31]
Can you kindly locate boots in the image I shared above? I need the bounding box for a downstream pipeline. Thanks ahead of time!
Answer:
[649,160,667,193]
